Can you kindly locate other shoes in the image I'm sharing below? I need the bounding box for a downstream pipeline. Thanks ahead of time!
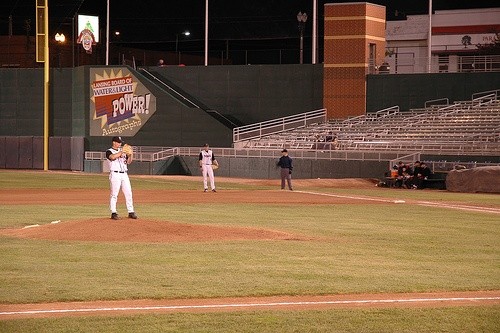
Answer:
[129,212,137,219]
[424,176,427,180]
[204,189,208,192]
[212,189,216,192]
[111,213,119,220]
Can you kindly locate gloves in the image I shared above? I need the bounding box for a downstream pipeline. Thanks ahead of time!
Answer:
[289,170,291,174]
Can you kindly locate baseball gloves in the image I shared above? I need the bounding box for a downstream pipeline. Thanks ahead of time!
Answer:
[122,144,133,156]
[212,164,218,169]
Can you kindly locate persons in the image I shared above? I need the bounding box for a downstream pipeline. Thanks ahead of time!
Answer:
[279,149,293,190]
[199,143,217,192]
[106,136,137,219]
[315,132,335,142]
[391,161,431,190]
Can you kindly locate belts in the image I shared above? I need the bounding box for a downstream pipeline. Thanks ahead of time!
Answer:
[114,171,124,173]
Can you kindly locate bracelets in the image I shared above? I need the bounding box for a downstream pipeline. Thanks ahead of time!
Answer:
[200,166,202,167]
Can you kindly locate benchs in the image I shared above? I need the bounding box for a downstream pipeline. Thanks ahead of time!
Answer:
[247,100,500,150]
[384,171,447,190]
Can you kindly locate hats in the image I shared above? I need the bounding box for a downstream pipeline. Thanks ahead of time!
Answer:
[204,144,208,147]
[112,136,124,143]
[281,149,287,152]
[399,161,404,164]
